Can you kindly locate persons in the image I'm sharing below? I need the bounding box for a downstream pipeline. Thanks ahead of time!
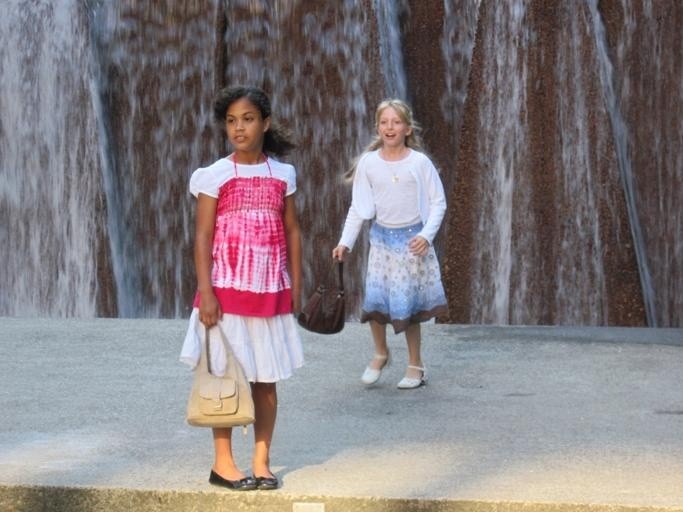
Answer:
[329,96,453,391]
[174,82,304,495]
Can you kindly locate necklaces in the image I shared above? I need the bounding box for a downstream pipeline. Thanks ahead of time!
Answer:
[381,150,404,185]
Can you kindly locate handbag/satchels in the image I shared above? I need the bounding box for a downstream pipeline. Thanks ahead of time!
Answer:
[298,258,347,335]
[187,322,254,429]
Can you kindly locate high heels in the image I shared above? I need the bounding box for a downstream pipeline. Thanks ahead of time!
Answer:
[360,351,391,384]
[397,364,427,389]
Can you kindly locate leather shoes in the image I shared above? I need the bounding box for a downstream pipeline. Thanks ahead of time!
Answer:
[209,470,278,492]
